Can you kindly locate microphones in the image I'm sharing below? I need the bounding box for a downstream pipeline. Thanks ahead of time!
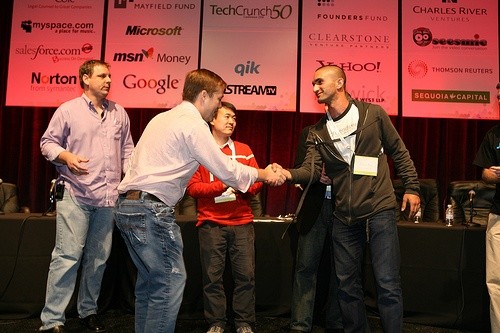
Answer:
[468,190,475,203]
[294,184,303,191]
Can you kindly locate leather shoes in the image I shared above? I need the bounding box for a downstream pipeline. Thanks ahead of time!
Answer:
[83,314,105,332]
[42,324,64,333]
[120,190,159,202]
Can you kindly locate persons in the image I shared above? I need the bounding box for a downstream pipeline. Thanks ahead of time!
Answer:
[471,81,500,333]
[37,59,134,333]
[263,65,421,333]
[187,101,263,333]
[112,68,286,333]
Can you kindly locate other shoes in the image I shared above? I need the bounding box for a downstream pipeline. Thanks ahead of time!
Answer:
[206,326,224,333]
[237,326,253,333]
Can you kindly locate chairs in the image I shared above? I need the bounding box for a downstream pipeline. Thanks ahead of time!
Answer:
[173,191,200,216]
[1,182,19,214]
[391,178,441,222]
[445,181,496,225]
[249,191,264,217]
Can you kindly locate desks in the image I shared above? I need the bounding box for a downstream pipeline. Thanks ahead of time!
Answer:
[0,212,492,330]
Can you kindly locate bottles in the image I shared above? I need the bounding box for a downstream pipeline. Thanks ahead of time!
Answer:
[414,208,422,223]
[445,204,454,227]
